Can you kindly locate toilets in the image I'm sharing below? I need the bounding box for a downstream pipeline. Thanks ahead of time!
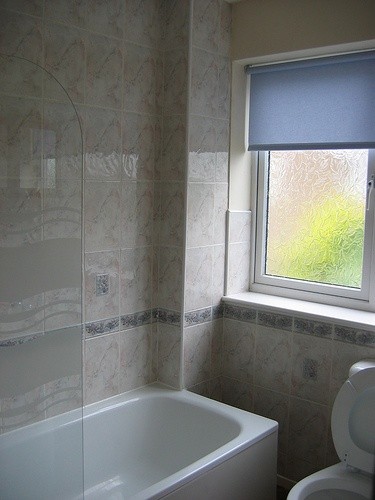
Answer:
[285,360,375,500]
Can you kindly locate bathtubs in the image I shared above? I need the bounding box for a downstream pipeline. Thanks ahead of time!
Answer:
[0,384,280,500]
